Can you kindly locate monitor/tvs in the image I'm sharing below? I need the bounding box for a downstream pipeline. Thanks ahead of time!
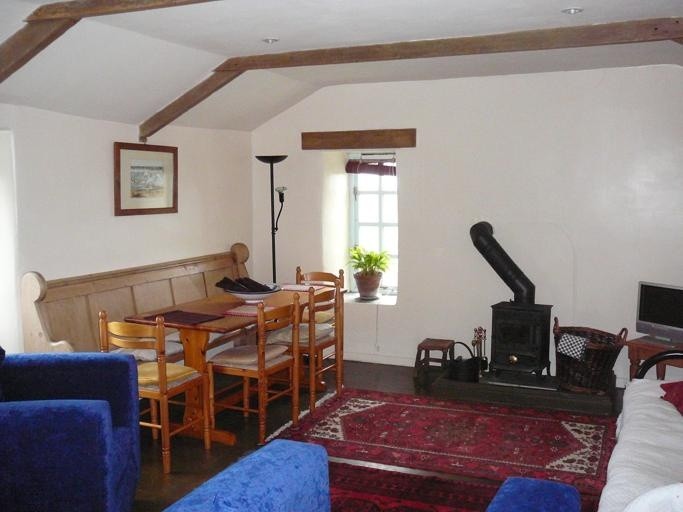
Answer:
[636,281,683,350]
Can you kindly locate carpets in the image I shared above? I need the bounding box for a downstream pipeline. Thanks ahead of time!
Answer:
[262,385,617,512]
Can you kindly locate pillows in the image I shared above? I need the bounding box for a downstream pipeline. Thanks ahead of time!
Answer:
[276,323,332,344]
[212,345,286,364]
[110,339,183,361]
[138,360,196,384]
[165,329,220,345]
[303,309,334,323]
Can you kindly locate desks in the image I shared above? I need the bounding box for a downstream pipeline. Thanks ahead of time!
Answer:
[624,336,683,381]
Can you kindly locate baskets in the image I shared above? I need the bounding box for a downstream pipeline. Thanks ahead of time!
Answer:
[552,317,629,396]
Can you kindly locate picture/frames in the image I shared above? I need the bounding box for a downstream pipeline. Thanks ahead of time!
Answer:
[112,142,179,217]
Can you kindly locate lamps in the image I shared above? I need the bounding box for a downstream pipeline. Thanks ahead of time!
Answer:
[256,155,289,324]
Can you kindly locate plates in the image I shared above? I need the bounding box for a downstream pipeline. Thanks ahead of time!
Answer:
[224,284,281,304]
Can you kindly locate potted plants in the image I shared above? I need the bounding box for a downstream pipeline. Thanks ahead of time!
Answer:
[347,246,387,298]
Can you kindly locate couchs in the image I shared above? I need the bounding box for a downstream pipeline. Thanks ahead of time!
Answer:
[21,242,278,402]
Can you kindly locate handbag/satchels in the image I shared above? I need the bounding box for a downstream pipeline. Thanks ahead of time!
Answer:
[445,342,481,384]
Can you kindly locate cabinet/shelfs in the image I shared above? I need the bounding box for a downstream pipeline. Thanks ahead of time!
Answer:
[491,307,549,371]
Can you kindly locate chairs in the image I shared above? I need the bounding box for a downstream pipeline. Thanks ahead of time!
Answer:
[278,280,343,411]
[296,266,343,322]
[207,294,301,445]
[0,345,141,511]
[162,440,580,512]
[99,310,210,474]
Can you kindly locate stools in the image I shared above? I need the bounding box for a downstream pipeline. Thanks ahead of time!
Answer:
[413,337,454,378]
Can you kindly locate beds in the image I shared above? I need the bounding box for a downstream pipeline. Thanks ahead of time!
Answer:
[600,351,683,512]
